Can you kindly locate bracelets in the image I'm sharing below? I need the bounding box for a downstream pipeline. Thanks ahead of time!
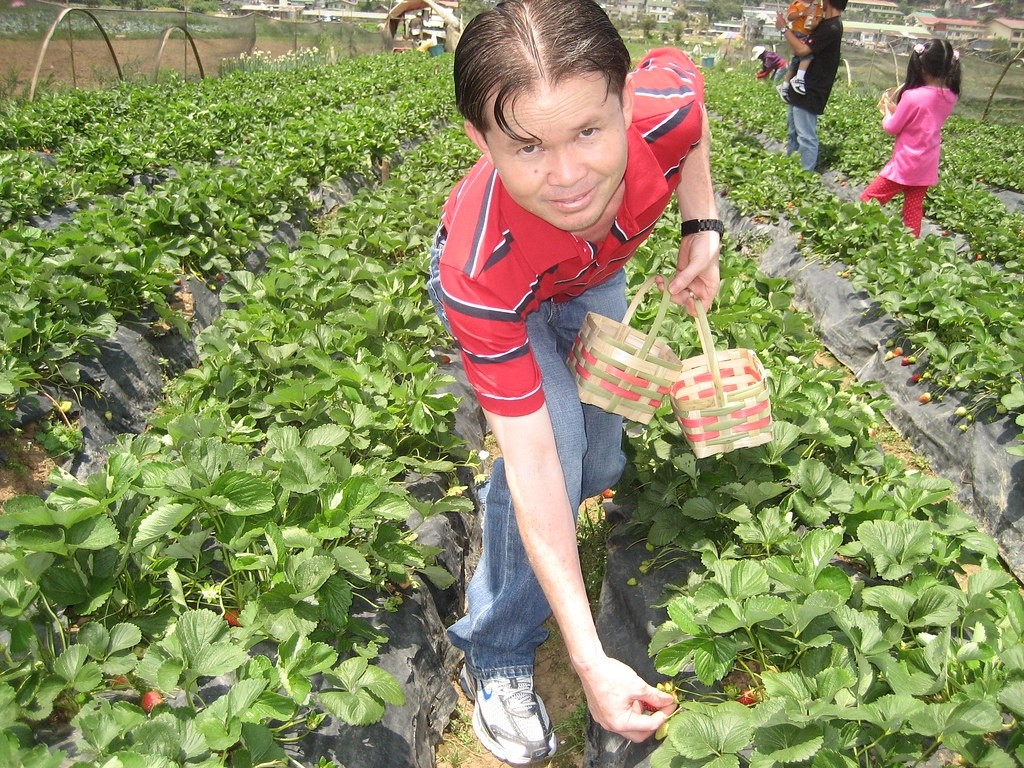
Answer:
[799,11,803,17]
[681,218,724,240]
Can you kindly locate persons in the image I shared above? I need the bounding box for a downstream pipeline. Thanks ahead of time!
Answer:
[430,0,721,765]
[750,46,789,81]
[776,0,823,103]
[859,39,961,239]
[775,0,848,172]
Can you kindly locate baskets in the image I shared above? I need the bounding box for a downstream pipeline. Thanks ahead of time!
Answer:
[669,296,774,460]
[876,82,906,117]
[565,273,684,426]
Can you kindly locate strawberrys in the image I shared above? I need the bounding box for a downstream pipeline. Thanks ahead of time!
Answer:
[882,338,972,433]
[141,690,164,713]
[604,486,759,741]
[223,609,242,627]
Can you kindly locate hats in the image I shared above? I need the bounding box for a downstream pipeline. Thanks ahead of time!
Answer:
[416,11,422,16]
[750,45,765,61]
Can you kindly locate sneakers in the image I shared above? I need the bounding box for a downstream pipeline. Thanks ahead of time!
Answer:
[459,660,557,765]
[775,84,790,104]
[789,77,806,95]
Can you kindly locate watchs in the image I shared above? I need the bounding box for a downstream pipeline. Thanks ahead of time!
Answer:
[781,26,789,34]
